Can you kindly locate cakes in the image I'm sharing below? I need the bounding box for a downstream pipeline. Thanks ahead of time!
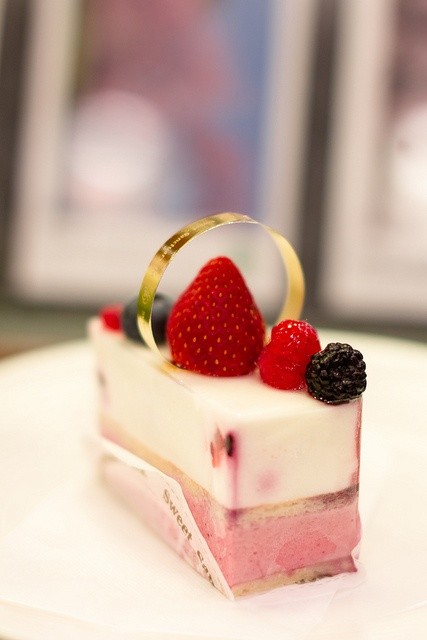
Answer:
[87,256,367,599]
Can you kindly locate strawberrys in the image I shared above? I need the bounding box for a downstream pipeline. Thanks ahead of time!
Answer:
[166,256,265,380]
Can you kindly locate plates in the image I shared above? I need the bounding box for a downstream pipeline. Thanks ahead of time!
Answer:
[2,329,427,640]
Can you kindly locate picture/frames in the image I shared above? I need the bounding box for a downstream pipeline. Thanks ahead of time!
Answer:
[317,1,426,346]
[2,0,320,321]
